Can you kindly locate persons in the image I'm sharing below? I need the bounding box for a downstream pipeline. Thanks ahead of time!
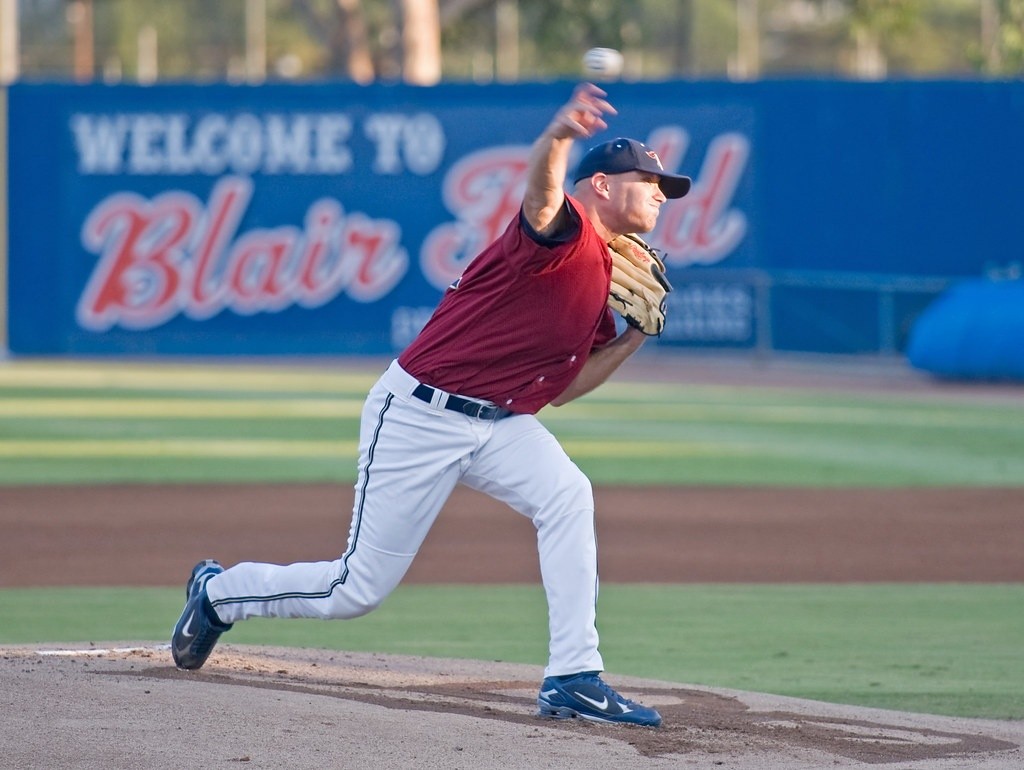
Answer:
[171,83,692,727]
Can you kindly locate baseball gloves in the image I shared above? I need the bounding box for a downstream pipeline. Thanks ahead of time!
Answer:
[610,225,673,339]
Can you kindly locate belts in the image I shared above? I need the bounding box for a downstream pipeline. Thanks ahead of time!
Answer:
[411,383,514,422]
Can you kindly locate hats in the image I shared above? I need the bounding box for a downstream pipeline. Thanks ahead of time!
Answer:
[571,138,692,199]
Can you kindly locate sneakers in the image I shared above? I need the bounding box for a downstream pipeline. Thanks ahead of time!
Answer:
[534,670,664,729]
[170,558,235,671]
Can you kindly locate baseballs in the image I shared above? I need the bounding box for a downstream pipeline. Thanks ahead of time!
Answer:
[580,47,627,81]
